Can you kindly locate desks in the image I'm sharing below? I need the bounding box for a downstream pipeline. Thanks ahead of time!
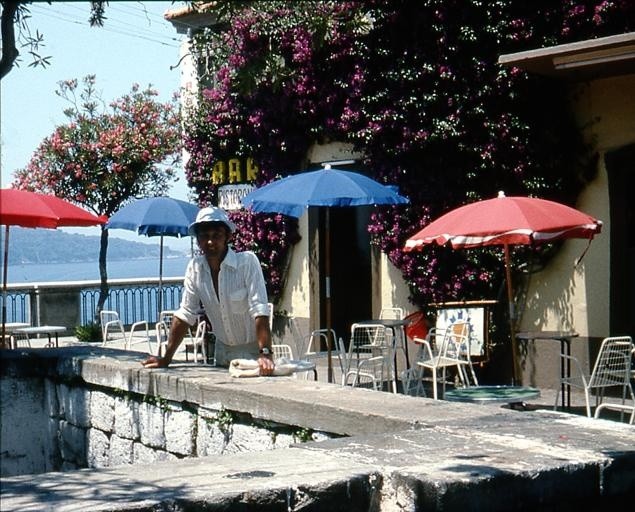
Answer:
[443,384,540,405]
[516,330,578,411]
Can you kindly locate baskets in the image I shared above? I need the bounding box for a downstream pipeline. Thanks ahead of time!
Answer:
[402,311,427,343]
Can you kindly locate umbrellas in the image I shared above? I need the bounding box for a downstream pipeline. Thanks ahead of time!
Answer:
[241,164,408,383]
[104,193,203,335]
[403,190,604,384]
[0,187,107,350]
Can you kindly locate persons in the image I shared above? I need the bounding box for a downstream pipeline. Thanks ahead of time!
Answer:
[140,202,275,375]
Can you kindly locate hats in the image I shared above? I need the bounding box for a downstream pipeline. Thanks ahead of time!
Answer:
[190,208,237,234]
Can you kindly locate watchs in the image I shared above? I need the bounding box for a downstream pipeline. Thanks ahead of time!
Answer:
[258,346,273,356]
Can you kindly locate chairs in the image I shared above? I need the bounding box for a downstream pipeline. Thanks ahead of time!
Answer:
[408,321,478,399]
[354,307,409,393]
[0,309,212,365]
[553,334,635,425]
[305,328,345,380]
[270,344,319,381]
[341,324,387,391]
